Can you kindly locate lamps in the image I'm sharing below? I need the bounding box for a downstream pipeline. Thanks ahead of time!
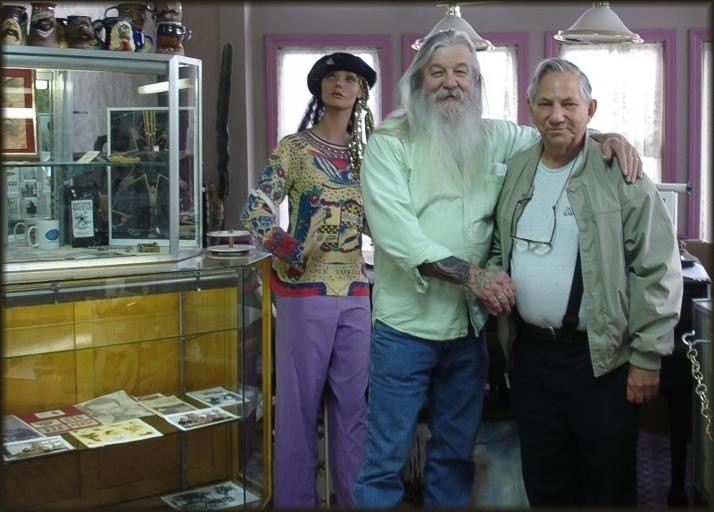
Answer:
[408,0,498,52]
[551,0,644,49]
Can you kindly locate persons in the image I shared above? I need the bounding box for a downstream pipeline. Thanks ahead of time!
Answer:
[236,51,380,510]
[355,27,645,509]
[484,55,684,510]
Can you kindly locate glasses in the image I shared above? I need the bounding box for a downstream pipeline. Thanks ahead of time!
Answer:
[509,197,557,257]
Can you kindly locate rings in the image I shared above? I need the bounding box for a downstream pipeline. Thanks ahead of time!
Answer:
[498,293,505,303]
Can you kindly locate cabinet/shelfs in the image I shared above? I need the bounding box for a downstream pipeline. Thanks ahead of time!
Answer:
[0,256,274,512]
[1,48,204,285]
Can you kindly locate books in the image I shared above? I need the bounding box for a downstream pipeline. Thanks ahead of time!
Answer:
[4,382,259,509]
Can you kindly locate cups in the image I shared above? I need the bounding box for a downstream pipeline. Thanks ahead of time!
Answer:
[27,219,60,251]
[13,217,48,244]
[56,0,191,54]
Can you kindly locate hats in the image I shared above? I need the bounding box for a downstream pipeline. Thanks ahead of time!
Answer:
[307,52,377,97]
